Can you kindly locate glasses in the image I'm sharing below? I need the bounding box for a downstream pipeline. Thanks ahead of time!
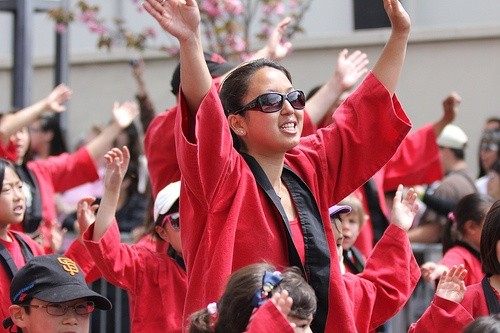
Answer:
[159,213,180,233]
[234,90,305,114]
[478,142,498,152]
[19,302,96,315]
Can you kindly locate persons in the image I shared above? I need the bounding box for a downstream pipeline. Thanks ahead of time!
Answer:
[0,159,100,332]
[187,261,317,333]
[141,0,413,333]
[81,146,192,333]
[3,255,113,333]
[292,49,500,333]
[145,17,370,215]
[1,82,159,246]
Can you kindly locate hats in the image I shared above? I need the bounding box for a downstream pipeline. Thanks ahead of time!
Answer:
[10,254,112,312]
[170,50,236,96]
[153,180,182,223]
[328,204,352,216]
[435,125,467,150]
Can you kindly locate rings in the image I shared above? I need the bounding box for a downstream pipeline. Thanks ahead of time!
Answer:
[159,0,170,6]
